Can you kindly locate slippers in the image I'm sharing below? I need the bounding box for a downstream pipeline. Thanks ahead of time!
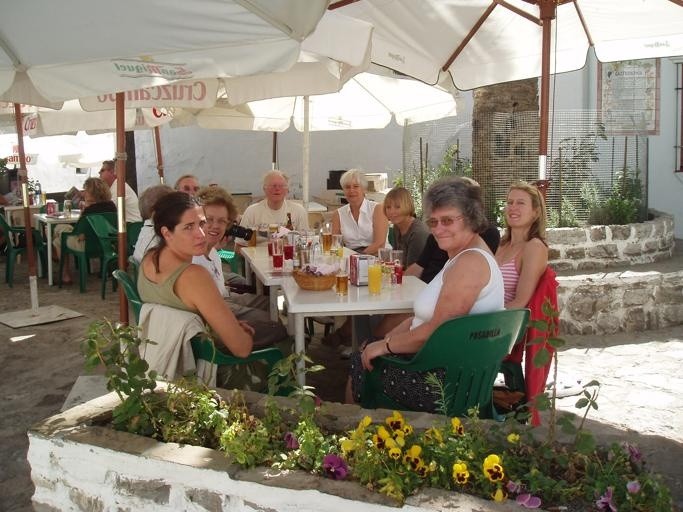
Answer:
[53,278,73,285]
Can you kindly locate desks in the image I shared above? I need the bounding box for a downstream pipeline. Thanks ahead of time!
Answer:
[4,199,58,265]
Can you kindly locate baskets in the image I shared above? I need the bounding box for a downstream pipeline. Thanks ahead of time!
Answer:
[293,269,336,290]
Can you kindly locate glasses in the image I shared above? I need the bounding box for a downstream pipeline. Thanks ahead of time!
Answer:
[426,215,465,228]
[184,186,201,191]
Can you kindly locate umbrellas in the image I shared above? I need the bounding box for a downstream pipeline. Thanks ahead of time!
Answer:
[302,0,683,204]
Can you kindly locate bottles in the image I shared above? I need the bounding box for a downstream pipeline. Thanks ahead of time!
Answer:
[25,178,71,222]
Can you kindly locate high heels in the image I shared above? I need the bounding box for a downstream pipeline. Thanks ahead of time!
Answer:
[321,330,352,347]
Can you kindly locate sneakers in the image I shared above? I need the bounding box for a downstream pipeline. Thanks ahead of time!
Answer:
[341,347,353,359]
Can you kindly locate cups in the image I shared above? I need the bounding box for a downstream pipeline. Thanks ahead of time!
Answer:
[268,220,404,297]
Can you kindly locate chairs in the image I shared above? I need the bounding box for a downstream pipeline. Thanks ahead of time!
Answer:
[128,218,143,242]
[1,215,48,288]
[126,300,289,398]
[108,268,140,312]
[495,266,555,423]
[38,202,77,270]
[217,246,241,277]
[86,216,135,298]
[216,193,390,239]
[358,310,528,418]
[54,211,116,296]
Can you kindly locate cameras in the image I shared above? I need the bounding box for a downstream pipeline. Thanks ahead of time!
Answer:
[224,224,254,241]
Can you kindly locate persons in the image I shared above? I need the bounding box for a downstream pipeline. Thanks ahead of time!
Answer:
[495,184,548,310]
[322,168,500,358]
[0,161,144,284]
[347,176,505,408]
[240,170,308,231]
[138,193,289,357]
[133,176,287,322]
